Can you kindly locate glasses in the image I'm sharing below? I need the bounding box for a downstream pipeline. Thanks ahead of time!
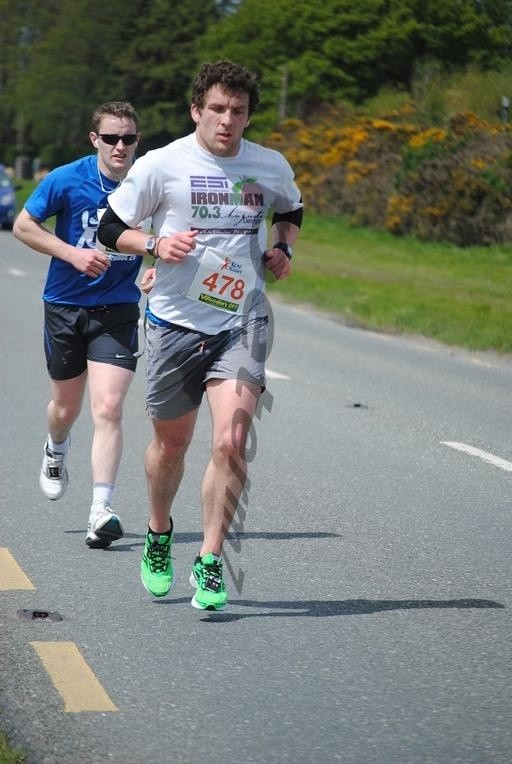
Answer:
[98,134,137,145]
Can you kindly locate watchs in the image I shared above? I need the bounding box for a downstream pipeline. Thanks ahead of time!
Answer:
[146,235,160,259]
[272,241,294,261]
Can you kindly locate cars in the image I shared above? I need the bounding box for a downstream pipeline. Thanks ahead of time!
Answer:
[0,167,24,230]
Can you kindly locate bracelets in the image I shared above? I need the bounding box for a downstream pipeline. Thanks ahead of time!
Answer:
[154,235,167,261]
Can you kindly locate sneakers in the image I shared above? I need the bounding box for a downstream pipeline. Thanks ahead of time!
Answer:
[189,552,228,611]
[140,517,174,597]
[85,501,126,548]
[39,438,69,500]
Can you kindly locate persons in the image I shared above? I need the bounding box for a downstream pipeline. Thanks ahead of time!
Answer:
[97,56,307,612]
[11,101,157,550]
